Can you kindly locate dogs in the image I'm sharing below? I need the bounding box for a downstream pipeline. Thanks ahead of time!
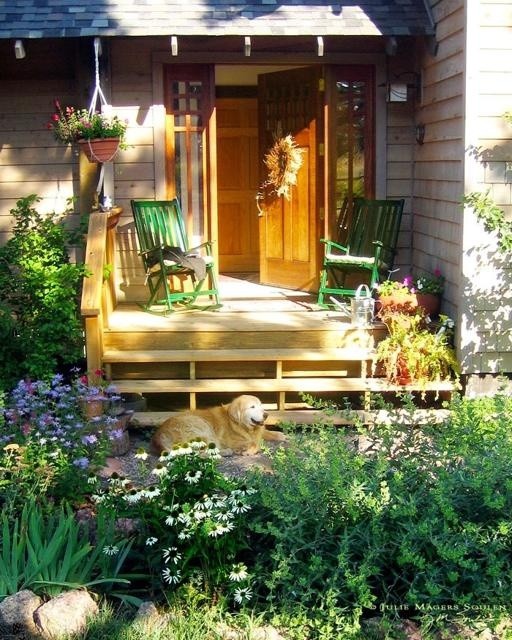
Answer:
[148,394,288,459]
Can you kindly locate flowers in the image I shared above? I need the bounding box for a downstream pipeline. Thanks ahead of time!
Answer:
[49,101,128,141]
[402,265,445,296]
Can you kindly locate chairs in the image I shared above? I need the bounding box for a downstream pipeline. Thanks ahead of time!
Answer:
[317,193,404,313]
[127,195,222,316]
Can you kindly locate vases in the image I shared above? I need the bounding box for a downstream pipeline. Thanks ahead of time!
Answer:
[411,293,442,320]
[78,135,119,164]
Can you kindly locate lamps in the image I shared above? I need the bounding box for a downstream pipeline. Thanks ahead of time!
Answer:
[376,71,424,104]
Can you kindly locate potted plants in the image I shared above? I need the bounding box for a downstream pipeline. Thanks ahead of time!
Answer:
[374,279,414,320]
[380,326,458,386]
